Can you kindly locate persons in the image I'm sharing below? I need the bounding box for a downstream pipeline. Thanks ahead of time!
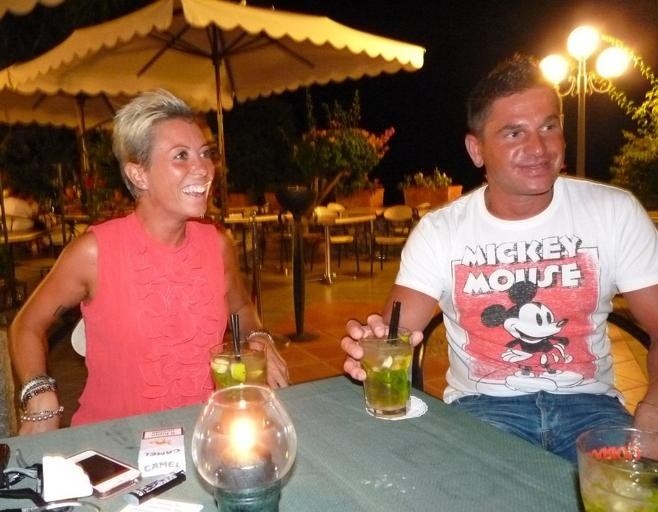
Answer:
[7,85,294,438]
[340,48,658,466]
[0,178,54,256]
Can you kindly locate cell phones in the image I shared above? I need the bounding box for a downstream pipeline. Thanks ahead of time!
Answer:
[61,447,143,502]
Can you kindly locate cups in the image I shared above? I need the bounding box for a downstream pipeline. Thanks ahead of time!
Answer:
[357,326,414,420]
[576,427,658,512]
[208,340,268,392]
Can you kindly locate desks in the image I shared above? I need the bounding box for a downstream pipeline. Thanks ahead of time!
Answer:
[0,372,577,512]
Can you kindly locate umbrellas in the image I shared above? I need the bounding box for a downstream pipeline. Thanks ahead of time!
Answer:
[1,0,428,221]
[2,88,140,188]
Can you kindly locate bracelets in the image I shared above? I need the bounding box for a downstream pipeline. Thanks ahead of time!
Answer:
[241,326,277,342]
[637,399,658,410]
[17,372,66,424]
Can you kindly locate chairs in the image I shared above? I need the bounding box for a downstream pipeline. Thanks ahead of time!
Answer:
[0,202,649,415]
